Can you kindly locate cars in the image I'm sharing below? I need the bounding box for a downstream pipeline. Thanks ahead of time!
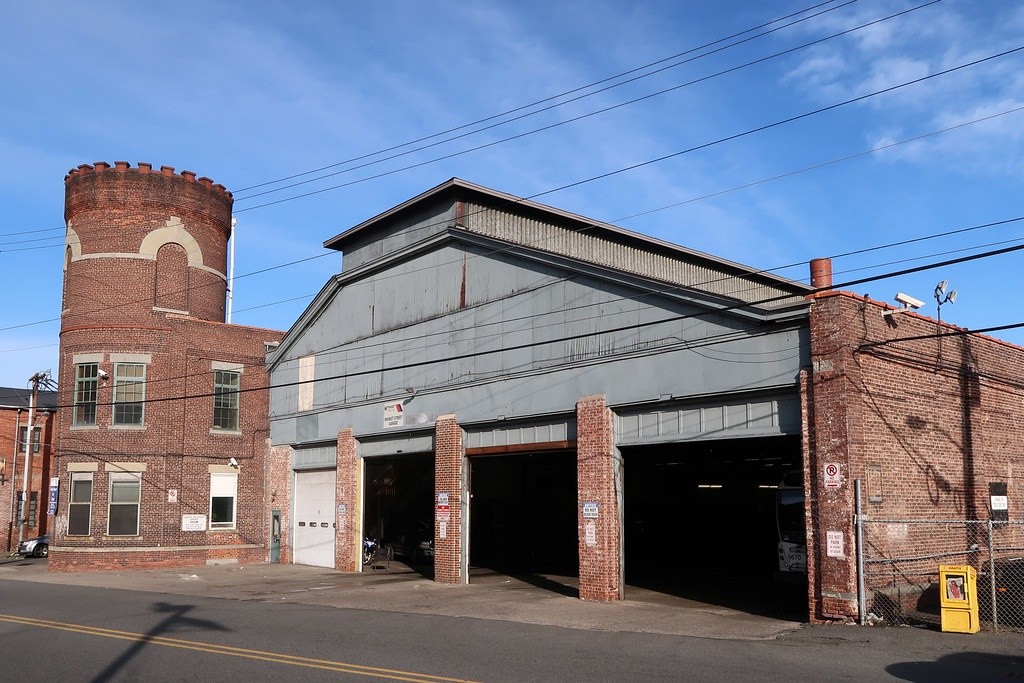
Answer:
[415,518,434,565]
[18,533,48,558]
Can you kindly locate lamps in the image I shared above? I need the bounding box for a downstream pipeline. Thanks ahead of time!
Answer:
[934,280,957,370]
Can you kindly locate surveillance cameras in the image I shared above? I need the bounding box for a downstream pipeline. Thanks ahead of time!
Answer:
[894,293,925,309]
[230,458,237,465]
[98,369,107,376]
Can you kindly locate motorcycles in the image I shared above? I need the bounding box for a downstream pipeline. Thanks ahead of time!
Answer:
[362,536,378,566]
